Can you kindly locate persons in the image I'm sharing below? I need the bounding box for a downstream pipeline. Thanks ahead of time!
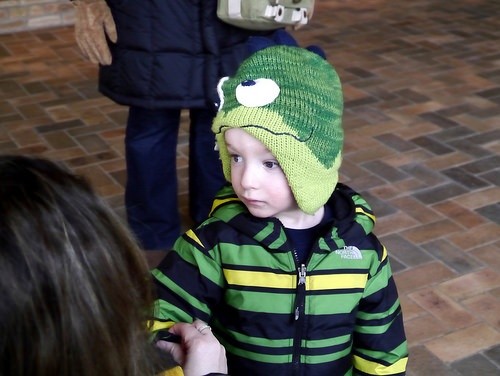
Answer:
[145,45,410,376]
[71,0,314,250]
[0,153,229,376]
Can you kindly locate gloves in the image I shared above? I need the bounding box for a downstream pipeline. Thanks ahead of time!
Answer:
[72,0,118,66]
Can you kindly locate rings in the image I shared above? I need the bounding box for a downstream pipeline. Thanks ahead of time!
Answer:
[199,326,209,332]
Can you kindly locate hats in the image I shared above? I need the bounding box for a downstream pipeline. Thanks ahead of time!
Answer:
[211,44,345,216]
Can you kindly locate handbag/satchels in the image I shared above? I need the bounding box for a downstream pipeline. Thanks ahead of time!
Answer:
[217,0,317,31]
